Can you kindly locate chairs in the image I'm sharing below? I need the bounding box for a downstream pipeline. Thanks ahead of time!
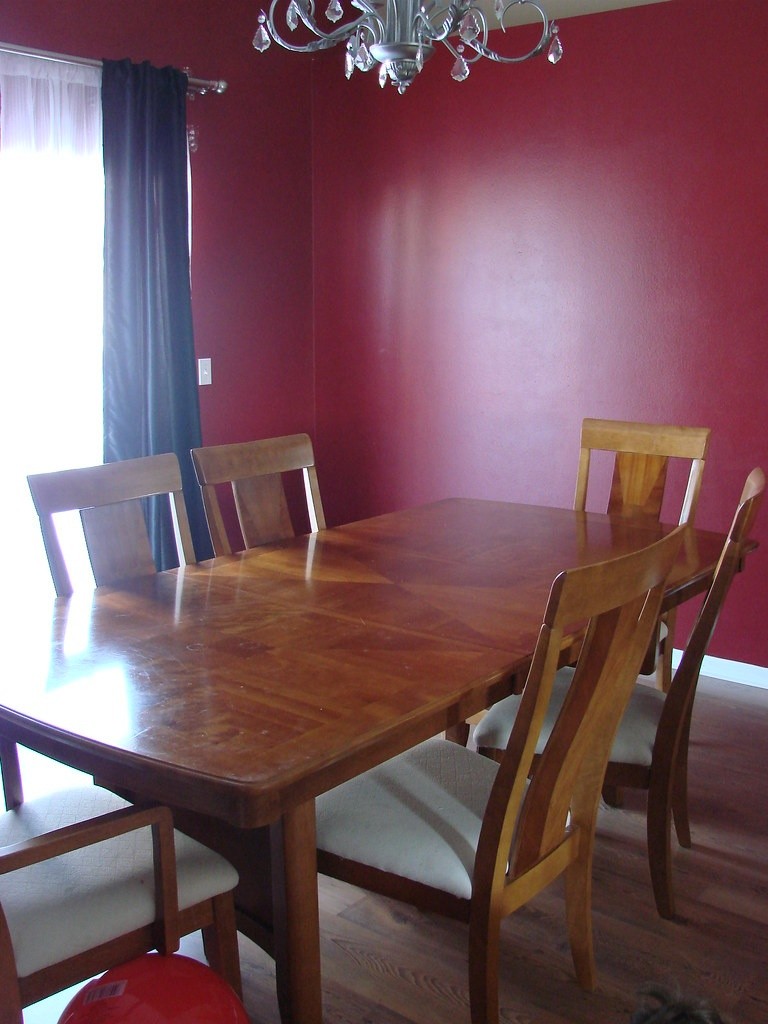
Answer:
[190,431,327,556]
[572,418,712,692]
[1,784,244,1024]
[467,466,766,919]
[29,451,198,599]
[305,520,690,1024]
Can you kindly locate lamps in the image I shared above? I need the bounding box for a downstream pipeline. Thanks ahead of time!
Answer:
[244,0,565,92]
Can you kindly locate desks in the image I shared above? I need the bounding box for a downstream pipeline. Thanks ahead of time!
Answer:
[1,498,765,1024]
